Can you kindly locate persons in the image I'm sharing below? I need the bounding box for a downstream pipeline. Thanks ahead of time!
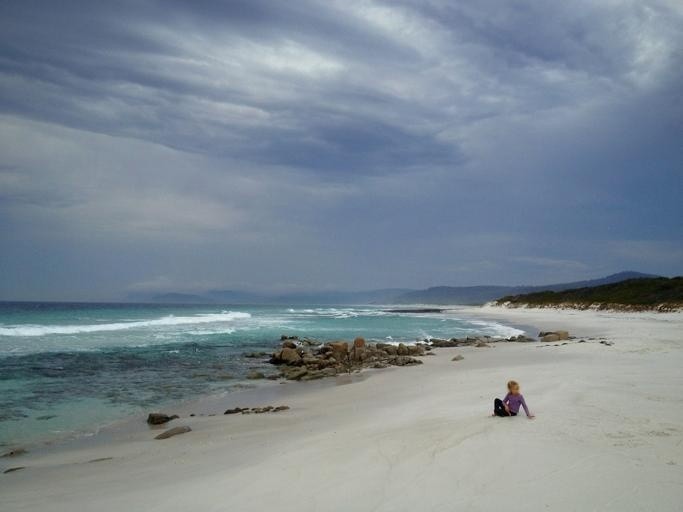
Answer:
[492,380,534,418]
